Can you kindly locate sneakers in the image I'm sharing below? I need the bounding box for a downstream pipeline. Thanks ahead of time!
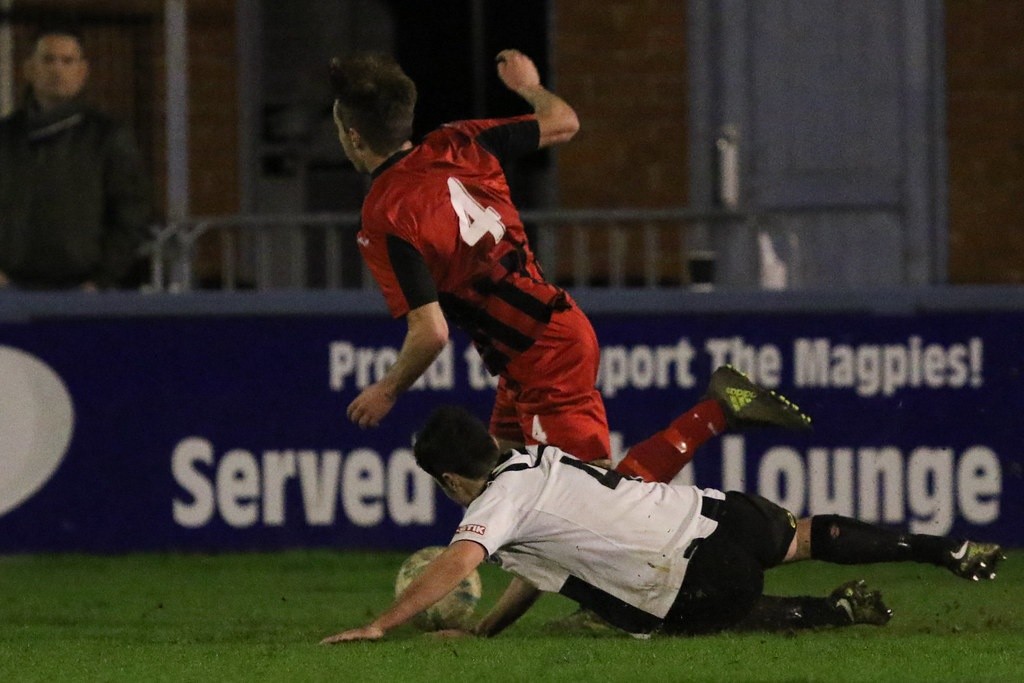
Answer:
[838,579,894,626]
[569,603,610,631]
[950,539,1002,582]
[698,363,811,432]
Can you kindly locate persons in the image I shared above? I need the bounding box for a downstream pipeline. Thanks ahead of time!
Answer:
[331,48,814,630]
[0,26,153,292]
[319,406,1007,645]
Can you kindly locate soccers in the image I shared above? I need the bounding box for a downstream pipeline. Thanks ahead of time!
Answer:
[394,546,482,632]
